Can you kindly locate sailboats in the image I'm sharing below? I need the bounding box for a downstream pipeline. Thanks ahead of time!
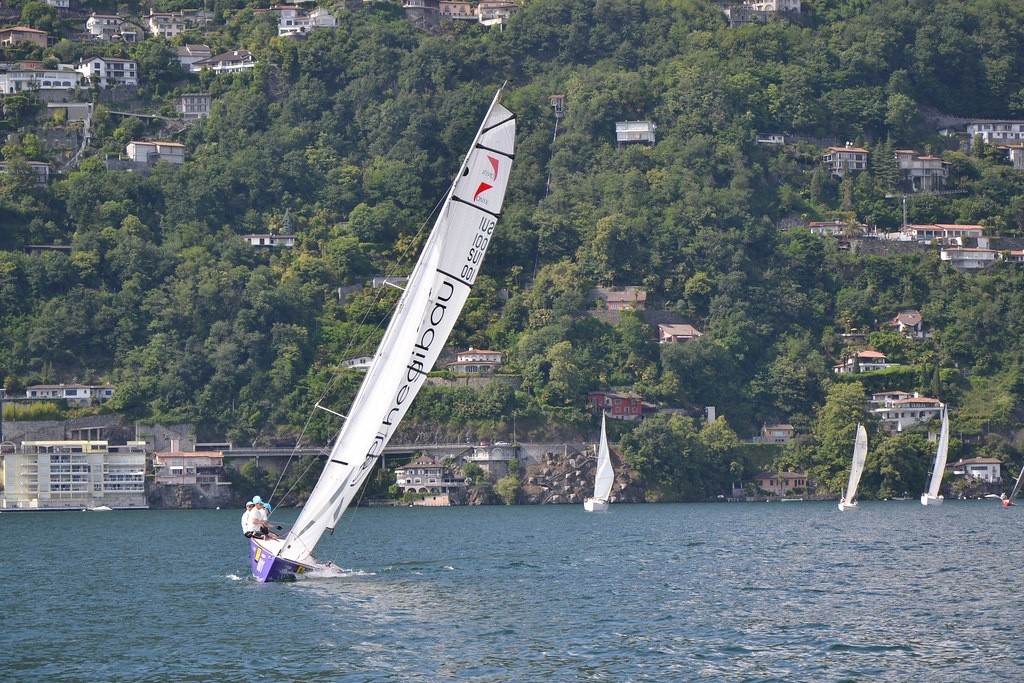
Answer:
[920,404,950,505]
[247,82,518,583]
[1002,466,1024,506]
[837,422,868,511]
[584,408,615,512]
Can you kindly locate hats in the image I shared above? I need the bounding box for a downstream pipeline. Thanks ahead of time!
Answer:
[262,503,272,513]
[246,500,254,507]
[252,495,265,505]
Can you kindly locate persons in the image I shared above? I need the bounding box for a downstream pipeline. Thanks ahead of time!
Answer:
[241,496,278,540]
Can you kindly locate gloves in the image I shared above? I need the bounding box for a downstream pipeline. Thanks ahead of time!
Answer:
[276,526,283,531]
